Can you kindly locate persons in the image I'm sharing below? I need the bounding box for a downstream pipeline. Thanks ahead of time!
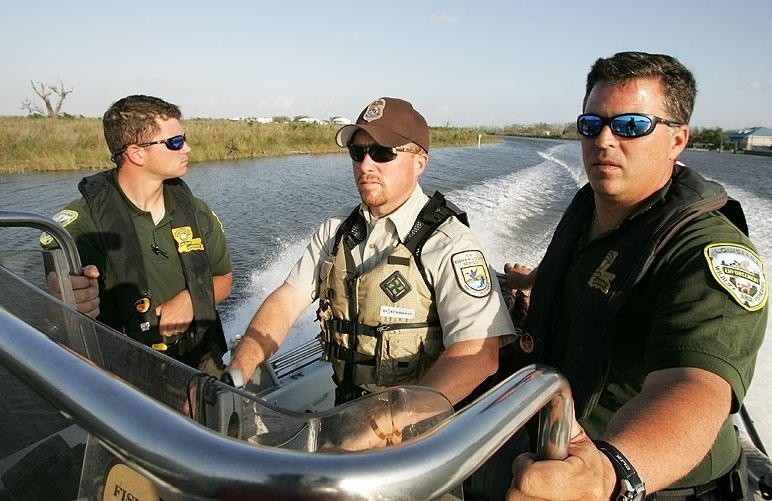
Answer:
[180,96,518,454]
[38,94,233,417]
[499,51,767,501]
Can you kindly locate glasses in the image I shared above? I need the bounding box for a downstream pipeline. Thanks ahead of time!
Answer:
[577,112,682,138]
[348,143,420,163]
[114,132,186,157]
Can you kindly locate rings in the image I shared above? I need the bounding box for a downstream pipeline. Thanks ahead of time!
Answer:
[173,329,181,334]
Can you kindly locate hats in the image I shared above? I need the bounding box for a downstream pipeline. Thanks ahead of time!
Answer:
[335,98,429,153]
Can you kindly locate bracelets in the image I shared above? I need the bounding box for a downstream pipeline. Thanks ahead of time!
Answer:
[369,414,403,447]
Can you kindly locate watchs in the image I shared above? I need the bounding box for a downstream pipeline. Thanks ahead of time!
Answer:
[592,439,646,501]
